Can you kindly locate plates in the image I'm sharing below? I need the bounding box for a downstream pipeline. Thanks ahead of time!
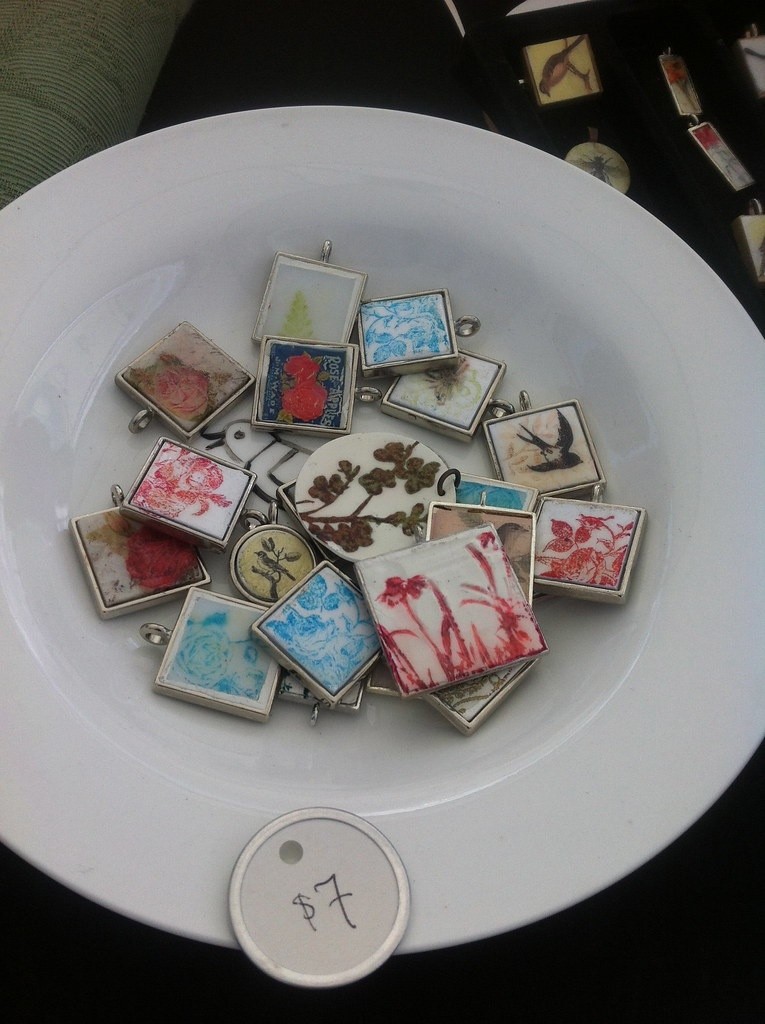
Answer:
[0,106,765,953]
